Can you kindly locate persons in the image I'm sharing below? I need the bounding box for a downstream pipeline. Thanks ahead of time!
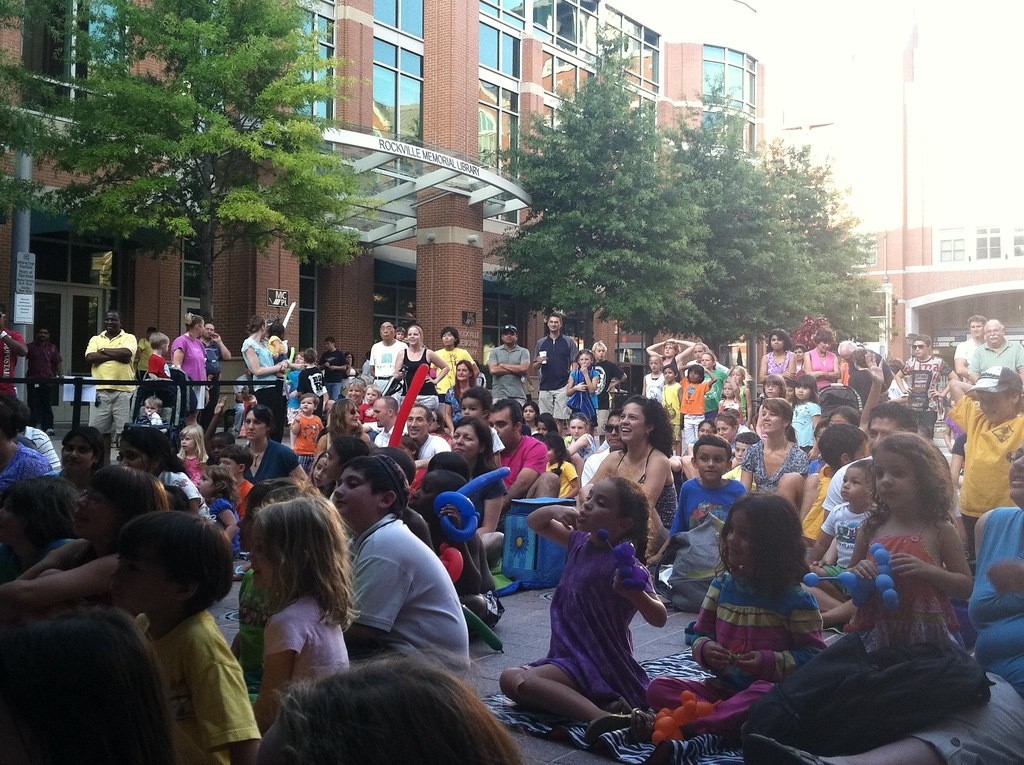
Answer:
[0,314,1024,765]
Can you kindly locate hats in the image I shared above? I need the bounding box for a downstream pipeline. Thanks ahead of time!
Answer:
[376,453,410,510]
[500,324,517,333]
[965,366,1023,395]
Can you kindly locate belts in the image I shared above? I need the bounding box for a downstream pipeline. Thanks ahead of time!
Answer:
[375,376,393,380]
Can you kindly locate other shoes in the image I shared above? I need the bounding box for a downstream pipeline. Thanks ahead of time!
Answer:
[46,427,56,435]
[584,713,631,744]
[742,734,823,765]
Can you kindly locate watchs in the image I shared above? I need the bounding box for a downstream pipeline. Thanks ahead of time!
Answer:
[0,331,7,339]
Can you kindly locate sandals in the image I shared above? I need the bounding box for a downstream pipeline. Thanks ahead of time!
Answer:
[630,707,656,744]
[232,561,250,581]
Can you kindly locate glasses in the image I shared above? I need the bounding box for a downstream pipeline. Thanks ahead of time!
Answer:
[79,489,111,506]
[64,444,92,453]
[38,331,49,335]
[346,409,360,415]
[117,451,143,461]
[250,403,269,415]
[605,425,621,433]
[911,344,925,351]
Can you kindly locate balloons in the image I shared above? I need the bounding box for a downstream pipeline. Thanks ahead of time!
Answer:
[388,363,428,448]
[652,691,722,745]
[281,302,295,328]
[285,346,294,383]
[597,529,648,590]
[432,467,510,542]
[461,604,504,654]
[439,543,464,583]
[803,542,900,609]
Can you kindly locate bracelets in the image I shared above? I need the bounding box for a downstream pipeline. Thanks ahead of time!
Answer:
[280,365,283,371]
[102,348,104,354]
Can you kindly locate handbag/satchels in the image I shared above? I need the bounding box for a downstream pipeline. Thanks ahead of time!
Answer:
[653,512,726,613]
